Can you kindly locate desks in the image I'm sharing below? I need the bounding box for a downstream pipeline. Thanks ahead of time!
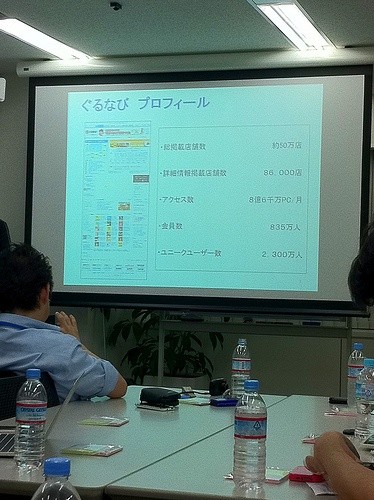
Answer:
[0,385,374,500]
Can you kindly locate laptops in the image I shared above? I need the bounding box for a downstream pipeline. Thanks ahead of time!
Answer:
[0,370,85,456]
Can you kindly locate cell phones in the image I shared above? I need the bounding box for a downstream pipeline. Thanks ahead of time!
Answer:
[45,314,61,326]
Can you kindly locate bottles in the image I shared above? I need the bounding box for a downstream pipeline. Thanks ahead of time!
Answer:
[14,369,47,471]
[347,343,367,408]
[355,359,374,440]
[31,457,81,500]
[233,380,267,490]
[232,338,251,400]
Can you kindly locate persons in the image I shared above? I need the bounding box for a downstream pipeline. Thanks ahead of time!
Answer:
[303,222,374,500]
[0,219,11,254]
[0,242,127,405]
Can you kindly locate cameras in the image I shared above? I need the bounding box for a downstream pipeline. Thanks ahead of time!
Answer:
[209,377,229,397]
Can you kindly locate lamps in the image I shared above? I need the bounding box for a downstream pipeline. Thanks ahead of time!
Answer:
[247,0,336,50]
[0,17,92,60]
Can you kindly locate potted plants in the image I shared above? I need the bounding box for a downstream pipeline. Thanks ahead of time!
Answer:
[90,308,252,389]
